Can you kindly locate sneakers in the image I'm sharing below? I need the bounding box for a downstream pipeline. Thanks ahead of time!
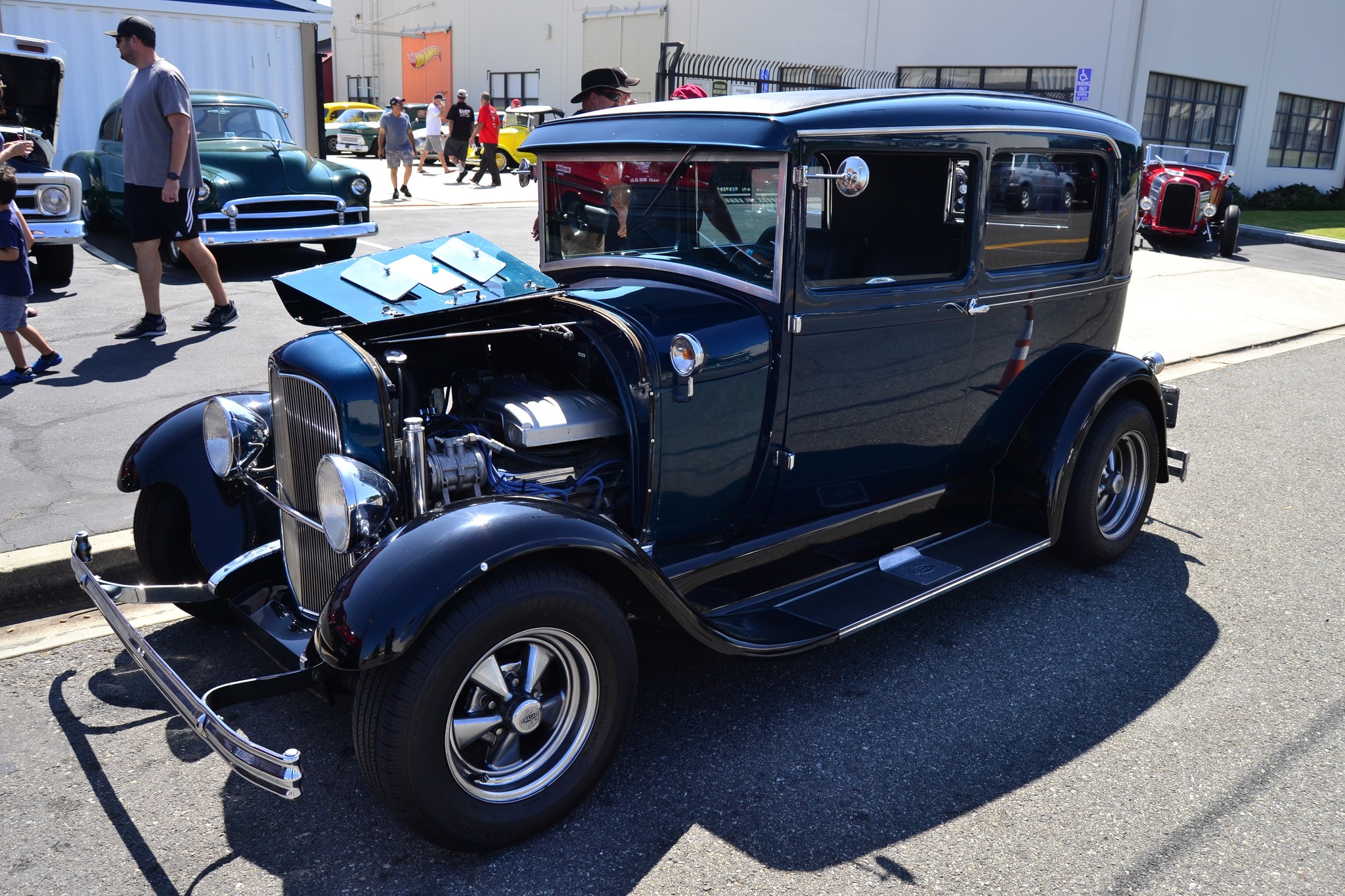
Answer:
[115,315,167,339]
[190,300,239,331]
[393,191,399,199]
[400,186,412,197]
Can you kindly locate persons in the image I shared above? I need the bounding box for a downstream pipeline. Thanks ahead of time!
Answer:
[378,96,418,199]
[531,67,640,258]
[621,83,744,251]
[417,93,456,174]
[446,89,475,183]
[0,73,37,318]
[0,166,62,385]
[103,15,237,337]
[502,98,521,129]
[469,92,501,186]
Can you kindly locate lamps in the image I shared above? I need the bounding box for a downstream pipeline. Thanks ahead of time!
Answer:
[355,14,360,20]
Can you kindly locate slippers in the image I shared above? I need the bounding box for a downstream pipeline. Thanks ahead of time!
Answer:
[417,170,429,173]
[469,179,479,185]
[488,184,502,186]
[445,170,455,173]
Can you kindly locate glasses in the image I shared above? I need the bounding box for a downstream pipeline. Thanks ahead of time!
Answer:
[514,103,517,107]
[596,91,622,101]
[394,103,405,106]
[0,84,7,90]
[115,37,123,44]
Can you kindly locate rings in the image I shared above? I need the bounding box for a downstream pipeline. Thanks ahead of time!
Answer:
[536,230,539,234]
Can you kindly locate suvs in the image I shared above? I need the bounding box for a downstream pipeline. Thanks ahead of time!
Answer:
[1052,154,1096,208]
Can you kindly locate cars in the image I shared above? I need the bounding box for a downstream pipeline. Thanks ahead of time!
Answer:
[464,104,566,173]
[0,31,86,289]
[324,102,383,123]
[324,108,384,155]
[68,87,1190,851]
[991,152,1075,211]
[335,103,447,159]
[1138,143,1241,257]
[62,87,379,271]
[409,110,534,167]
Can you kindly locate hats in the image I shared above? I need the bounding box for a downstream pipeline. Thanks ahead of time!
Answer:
[605,65,641,86]
[512,99,521,107]
[390,96,405,105]
[480,92,491,100]
[434,93,446,101]
[670,83,707,100]
[457,89,468,97]
[104,15,158,41]
[571,67,633,104]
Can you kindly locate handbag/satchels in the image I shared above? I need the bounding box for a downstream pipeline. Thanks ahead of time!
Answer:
[559,189,612,235]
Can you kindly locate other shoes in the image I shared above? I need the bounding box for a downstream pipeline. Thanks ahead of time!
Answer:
[456,170,468,182]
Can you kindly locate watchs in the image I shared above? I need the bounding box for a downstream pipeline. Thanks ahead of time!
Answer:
[167,172,181,180]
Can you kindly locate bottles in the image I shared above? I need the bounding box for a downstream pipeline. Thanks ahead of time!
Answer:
[471,142,478,152]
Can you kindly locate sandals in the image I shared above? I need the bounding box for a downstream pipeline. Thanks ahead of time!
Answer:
[24,305,37,317]
[0,369,35,385]
[30,349,63,374]
